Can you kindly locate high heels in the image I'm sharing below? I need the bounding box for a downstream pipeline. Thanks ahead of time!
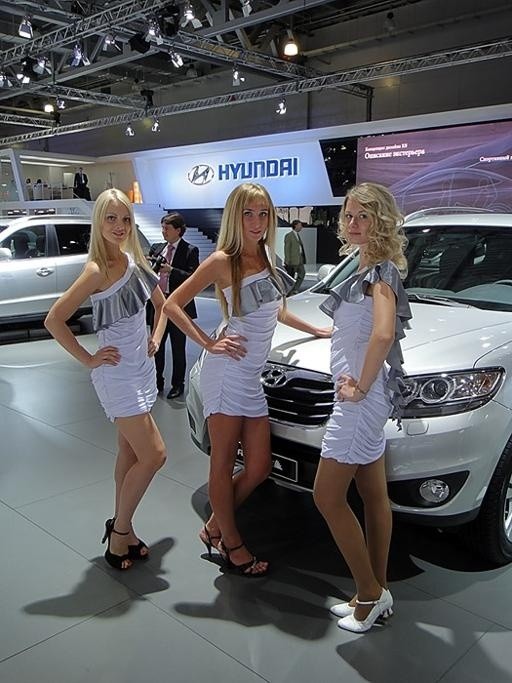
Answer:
[199,523,223,559]
[330,585,395,634]
[215,537,270,579]
[101,516,150,571]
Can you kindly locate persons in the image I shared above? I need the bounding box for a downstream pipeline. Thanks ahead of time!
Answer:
[145,213,199,398]
[25,178,33,185]
[44,185,173,570]
[313,181,411,636]
[282,219,307,292]
[72,166,89,196]
[164,183,338,579]
[35,177,49,188]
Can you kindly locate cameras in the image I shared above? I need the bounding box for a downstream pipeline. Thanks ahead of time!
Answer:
[150,255,167,274]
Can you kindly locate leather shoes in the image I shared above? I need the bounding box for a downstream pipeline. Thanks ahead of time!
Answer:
[157,386,164,396]
[166,383,185,400]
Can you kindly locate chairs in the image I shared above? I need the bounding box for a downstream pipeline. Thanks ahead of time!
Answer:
[24,182,76,201]
[422,244,485,293]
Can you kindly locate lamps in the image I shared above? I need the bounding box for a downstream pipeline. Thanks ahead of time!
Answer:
[102,34,123,58]
[232,60,245,89]
[127,32,151,54]
[19,15,34,38]
[51,112,62,130]
[169,44,184,70]
[152,118,161,133]
[56,98,67,111]
[284,38,299,56]
[43,136,51,153]
[43,100,55,115]
[125,122,135,136]
[383,4,395,31]
[128,66,145,91]
[147,1,253,41]
[141,89,154,108]
[73,42,94,69]
[185,63,197,80]
[2,49,54,92]
[275,94,287,117]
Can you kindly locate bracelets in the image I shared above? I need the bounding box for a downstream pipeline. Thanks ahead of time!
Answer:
[356,383,369,394]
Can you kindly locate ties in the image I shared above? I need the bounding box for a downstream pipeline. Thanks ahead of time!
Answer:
[80,173,83,183]
[158,244,175,295]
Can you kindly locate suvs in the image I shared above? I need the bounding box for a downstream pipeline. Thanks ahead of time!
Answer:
[1,213,152,330]
[185,205,512,562]
